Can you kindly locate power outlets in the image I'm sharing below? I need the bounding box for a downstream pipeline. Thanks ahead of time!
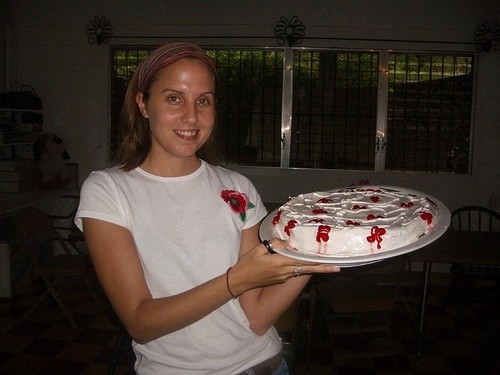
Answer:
[490,195,500,208]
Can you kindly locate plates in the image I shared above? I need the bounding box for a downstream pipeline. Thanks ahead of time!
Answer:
[258,185,451,267]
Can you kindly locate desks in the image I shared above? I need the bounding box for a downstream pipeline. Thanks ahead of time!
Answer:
[0,192,33,220]
[405,231,500,363]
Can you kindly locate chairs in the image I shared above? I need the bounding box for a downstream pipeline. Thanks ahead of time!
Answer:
[274,206,500,375]
[13,207,126,375]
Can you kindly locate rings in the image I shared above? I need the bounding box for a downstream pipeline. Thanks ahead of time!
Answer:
[296,272,302,276]
[263,240,273,252]
[294,266,301,273]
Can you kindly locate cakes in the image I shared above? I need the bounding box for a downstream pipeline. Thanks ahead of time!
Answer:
[273,185,439,257]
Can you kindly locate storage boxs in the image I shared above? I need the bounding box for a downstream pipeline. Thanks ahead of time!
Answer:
[0,232,34,299]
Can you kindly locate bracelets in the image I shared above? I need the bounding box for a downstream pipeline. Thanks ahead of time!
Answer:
[227,267,238,299]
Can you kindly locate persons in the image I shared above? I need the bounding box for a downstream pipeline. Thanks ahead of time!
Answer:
[72,41,340,375]
